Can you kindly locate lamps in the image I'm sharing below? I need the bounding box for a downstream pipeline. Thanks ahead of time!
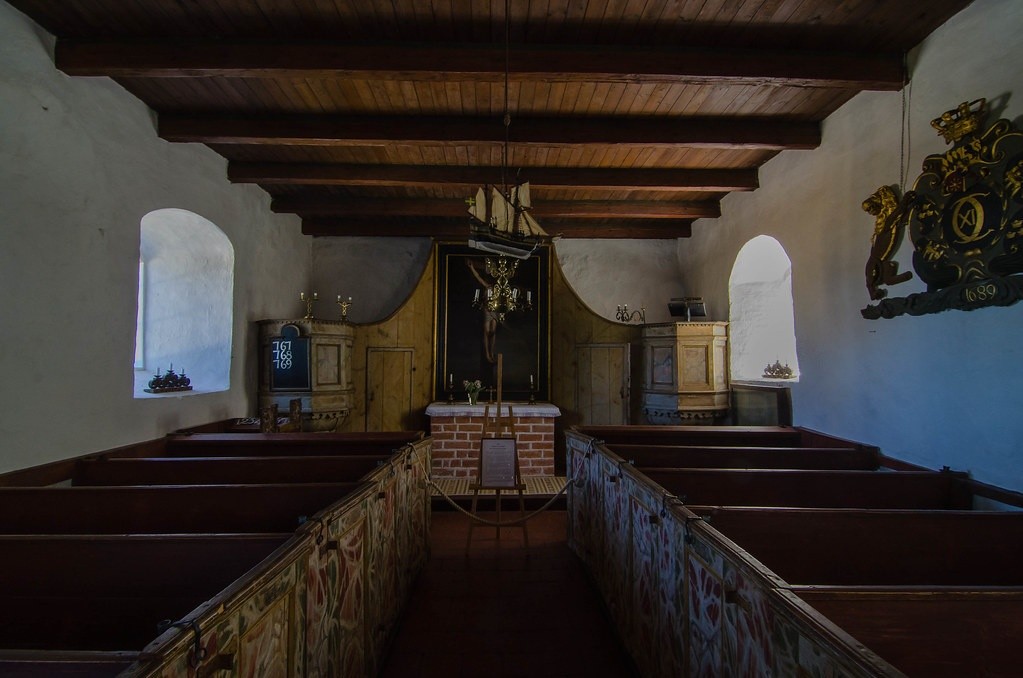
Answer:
[337,294,352,321]
[300,292,320,319]
[470,138,534,323]
[615,303,646,323]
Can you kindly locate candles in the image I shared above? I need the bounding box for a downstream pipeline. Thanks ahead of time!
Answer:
[450,373,453,382]
[530,374,533,382]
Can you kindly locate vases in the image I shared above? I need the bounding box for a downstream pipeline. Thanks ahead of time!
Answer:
[468,392,478,405]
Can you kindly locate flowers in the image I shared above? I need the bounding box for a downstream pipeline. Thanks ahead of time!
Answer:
[462,379,483,404]
